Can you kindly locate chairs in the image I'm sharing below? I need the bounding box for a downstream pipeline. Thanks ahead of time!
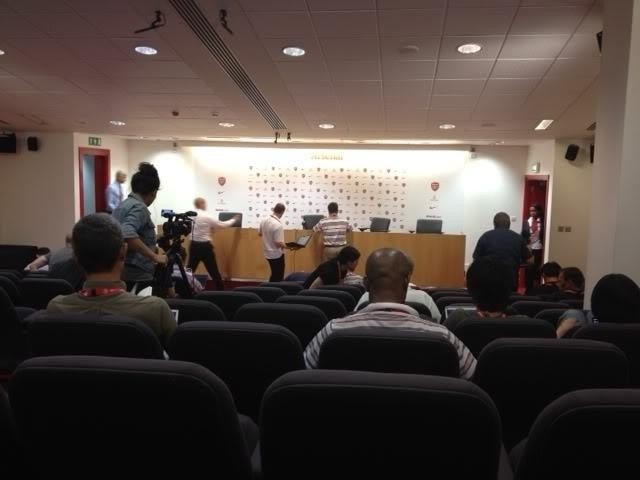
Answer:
[146,298,224,324]
[19,313,164,362]
[19,277,77,309]
[2,243,38,267]
[316,286,361,303]
[454,316,554,357]
[416,219,442,233]
[479,338,626,448]
[506,295,541,307]
[510,299,572,317]
[359,218,390,232]
[509,388,640,479]
[259,367,504,479]
[203,289,263,319]
[438,297,495,322]
[237,305,329,351]
[577,322,639,362]
[300,286,355,311]
[0,276,17,290]
[258,281,302,295]
[172,323,302,426]
[426,287,464,301]
[2,356,250,480]
[235,287,286,303]
[0,286,15,315]
[317,327,460,379]
[276,296,352,322]
[433,292,469,302]
[537,309,594,323]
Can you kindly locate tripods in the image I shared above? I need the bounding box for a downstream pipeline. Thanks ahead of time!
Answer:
[157,244,195,301]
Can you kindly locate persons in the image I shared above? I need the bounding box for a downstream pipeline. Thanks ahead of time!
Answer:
[303,245,360,289]
[258,201,301,282]
[525,260,561,296]
[549,266,585,301]
[443,251,527,328]
[302,247,478,380]
[22,162,243,357]
[311,202,354,259]
[470,210,535,292]
[522,204,544,295]
[352,249,442,324]
[558,273,640,337]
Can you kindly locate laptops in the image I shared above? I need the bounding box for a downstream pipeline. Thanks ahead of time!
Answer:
[170,308,179,324]
[370,218,390,233]
[445,306,477,320]
[286,234,312,249]
[416,219,442,235]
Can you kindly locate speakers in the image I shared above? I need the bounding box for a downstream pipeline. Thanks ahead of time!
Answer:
[590,144,594,164]
[565,144,579,161]
[1,131,16,153]
[28,137,38,151]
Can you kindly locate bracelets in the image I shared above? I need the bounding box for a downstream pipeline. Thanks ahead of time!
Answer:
[290,247,293,251]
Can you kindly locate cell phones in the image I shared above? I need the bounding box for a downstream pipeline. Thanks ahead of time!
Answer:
[592,317,598,325]
